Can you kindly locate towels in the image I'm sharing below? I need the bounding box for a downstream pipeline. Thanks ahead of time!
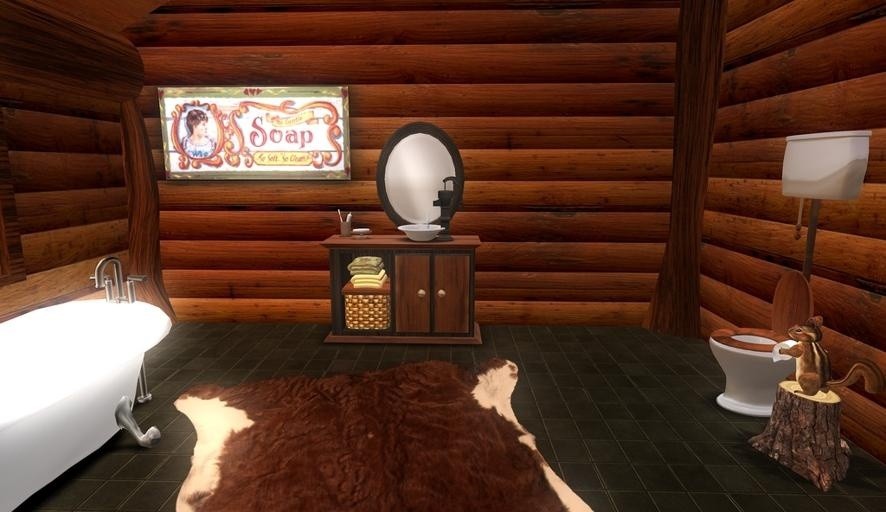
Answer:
[346,256,387,292]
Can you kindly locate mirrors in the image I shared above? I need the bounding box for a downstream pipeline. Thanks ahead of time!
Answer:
[376,122,465,242]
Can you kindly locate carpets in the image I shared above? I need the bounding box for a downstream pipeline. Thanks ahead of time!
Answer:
[174,359,593,512]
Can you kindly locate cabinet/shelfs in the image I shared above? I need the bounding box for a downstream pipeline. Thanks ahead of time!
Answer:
[323,233,482,347]
